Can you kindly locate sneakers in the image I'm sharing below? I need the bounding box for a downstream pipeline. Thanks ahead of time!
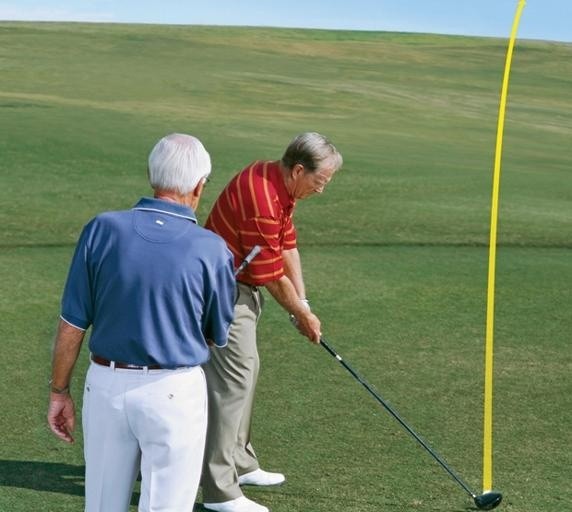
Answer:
[238,468,285,486]
[204,495,268,512]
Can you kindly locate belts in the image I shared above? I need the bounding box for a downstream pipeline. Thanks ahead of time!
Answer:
[289,300,311,329]
[91,356,160,370]
[236,280,257,291]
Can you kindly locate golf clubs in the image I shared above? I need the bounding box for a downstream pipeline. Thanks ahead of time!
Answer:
[289,313,502,511]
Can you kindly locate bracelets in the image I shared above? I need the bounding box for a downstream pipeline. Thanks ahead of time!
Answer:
[49,384,70,394]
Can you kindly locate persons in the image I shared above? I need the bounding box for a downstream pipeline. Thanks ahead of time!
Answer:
[47,134,236,512]
[201,132,343,512]
